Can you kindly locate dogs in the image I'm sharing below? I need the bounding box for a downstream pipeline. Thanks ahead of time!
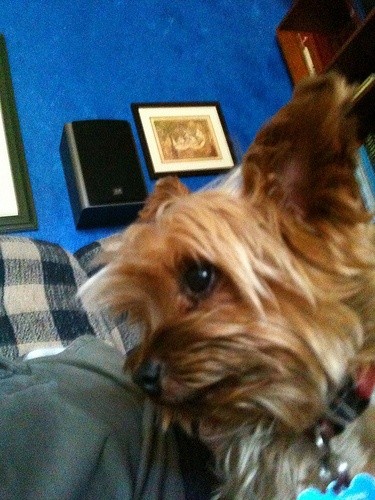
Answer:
[80,73,375,500]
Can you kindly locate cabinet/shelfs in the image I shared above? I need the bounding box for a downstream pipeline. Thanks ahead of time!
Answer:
[276,0,375,155]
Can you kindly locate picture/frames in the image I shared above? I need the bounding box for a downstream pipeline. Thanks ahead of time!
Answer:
[132,103,237,179]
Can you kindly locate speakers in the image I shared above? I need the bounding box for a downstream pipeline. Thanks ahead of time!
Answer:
[59,120,149,231]
